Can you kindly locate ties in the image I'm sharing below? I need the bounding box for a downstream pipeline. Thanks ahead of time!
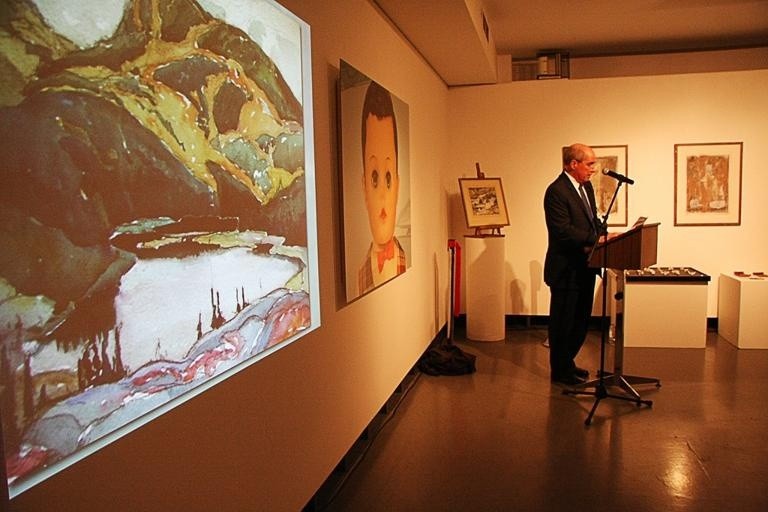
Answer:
[578,183,593,222]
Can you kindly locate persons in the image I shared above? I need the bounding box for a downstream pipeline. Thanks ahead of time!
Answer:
[358,80,407,296]
[543,143,624,385]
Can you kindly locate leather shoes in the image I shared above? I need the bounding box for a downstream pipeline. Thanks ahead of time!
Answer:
[575,368,588,377]
[551,374,585,385]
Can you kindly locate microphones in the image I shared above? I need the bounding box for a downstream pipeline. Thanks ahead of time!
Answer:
[602,167,634,186]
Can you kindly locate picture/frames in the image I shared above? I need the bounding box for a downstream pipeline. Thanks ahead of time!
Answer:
[459,178,510,228]
[563,146,628,227]
[674,142,742,225]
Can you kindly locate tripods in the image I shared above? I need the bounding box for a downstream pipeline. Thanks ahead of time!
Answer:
[562,224,654,426]
